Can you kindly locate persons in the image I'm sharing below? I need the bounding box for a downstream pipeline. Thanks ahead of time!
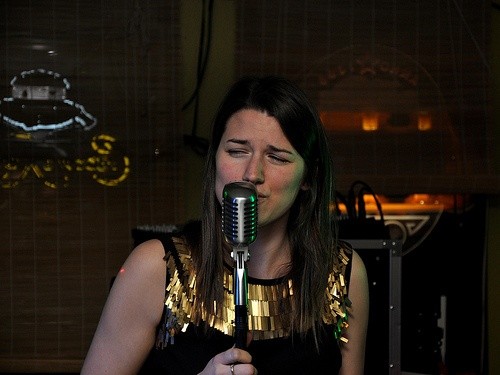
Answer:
[79,71,370,375]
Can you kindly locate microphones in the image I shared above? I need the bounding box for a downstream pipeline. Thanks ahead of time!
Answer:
[221,182,259,306]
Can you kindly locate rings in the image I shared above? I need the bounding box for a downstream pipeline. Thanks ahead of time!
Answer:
[230,364,234,375]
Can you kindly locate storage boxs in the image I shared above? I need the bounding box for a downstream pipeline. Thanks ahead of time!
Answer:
[342,239,401,375]
